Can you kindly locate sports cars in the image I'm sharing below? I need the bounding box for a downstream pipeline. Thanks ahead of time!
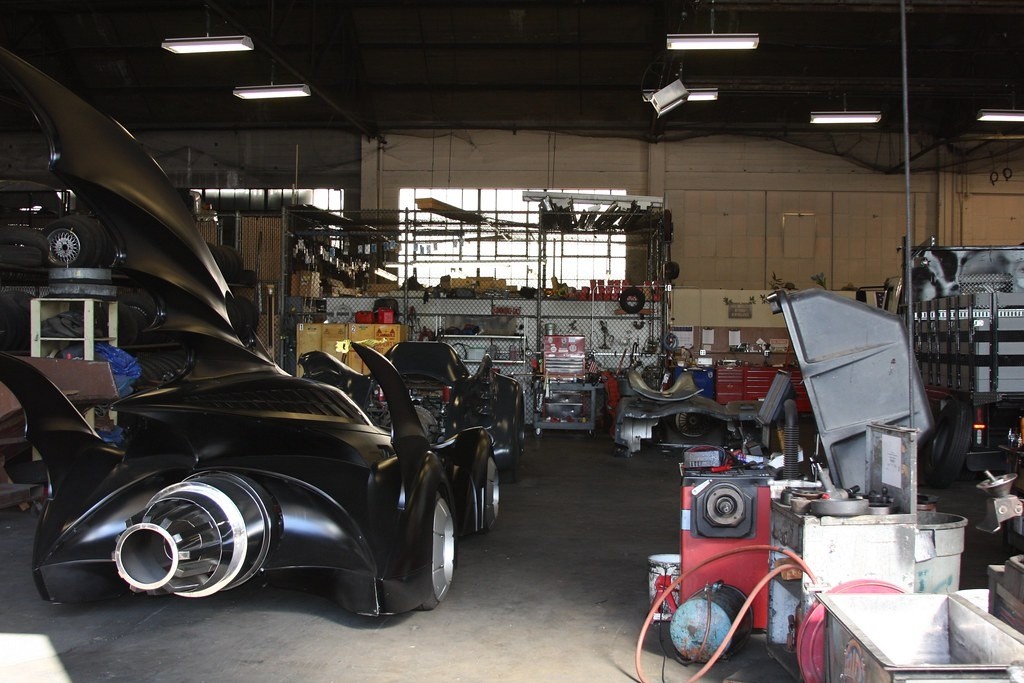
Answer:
[296,341,525,483]
[0,49,501,618]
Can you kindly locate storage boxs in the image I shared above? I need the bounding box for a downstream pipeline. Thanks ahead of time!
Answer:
[291,267,400,300]
[544,334,586,383]
[295,323,408,378]
[440,275,518,298]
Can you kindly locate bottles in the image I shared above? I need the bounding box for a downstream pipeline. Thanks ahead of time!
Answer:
[822,486,861,500]
[1008,426,1022,449]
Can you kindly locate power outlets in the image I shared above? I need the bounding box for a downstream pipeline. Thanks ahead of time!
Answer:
[699,350,707,356]
[764,350,770,356]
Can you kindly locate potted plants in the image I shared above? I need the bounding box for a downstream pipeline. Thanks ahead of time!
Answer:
[722,294,766,319]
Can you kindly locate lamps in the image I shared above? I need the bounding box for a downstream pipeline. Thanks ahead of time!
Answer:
[666,8,760,51]
[233,60,311,100]
[160,7,256,55]
[975,97,1024,122]
[810,93,882,124]
[635,62,720,118]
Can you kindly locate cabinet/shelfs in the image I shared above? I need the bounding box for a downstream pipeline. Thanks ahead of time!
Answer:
[530,379,598,435]
[766,499,804,683]
[280,299,670,435]
[29,297,119,463]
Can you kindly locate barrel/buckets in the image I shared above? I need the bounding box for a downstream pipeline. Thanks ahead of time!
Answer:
[648,554,681,619]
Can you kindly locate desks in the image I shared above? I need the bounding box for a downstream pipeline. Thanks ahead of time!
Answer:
[674,366,714,402]
[716,361,813,414]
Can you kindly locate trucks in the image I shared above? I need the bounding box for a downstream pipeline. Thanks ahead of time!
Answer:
[854,236,1023,484]
